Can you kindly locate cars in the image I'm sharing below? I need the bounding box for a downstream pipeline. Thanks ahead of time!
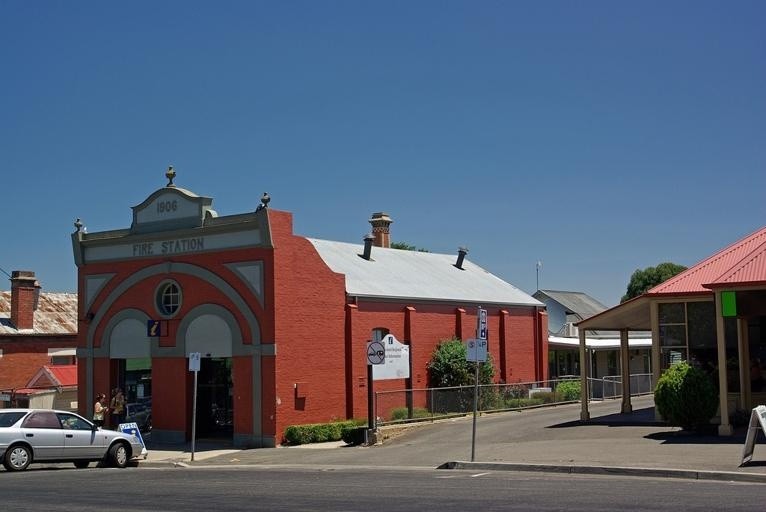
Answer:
[123,403,151,432]
[0,408,144,472]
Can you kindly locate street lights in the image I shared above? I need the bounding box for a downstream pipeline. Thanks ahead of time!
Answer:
[536,259,543,299]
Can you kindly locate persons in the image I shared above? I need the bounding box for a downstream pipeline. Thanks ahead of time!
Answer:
[110,387,126,425]
[92,392,109,427]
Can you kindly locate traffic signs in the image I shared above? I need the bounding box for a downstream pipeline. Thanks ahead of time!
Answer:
[481,310,486,339]
